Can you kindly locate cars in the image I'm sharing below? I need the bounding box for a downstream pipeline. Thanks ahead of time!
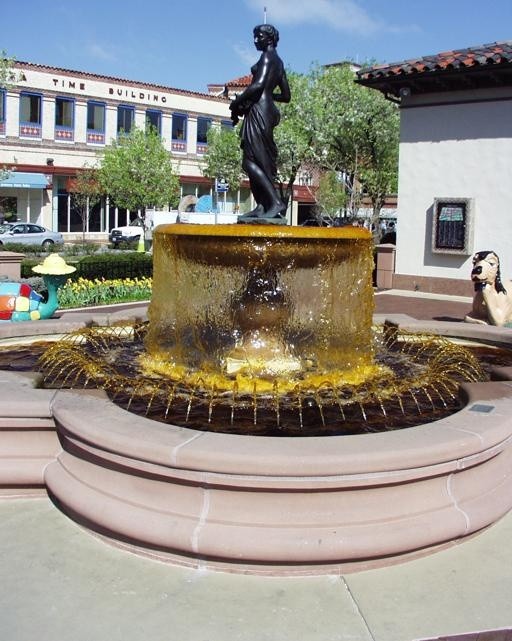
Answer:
[0,222,64,251]
[109,218,144,245]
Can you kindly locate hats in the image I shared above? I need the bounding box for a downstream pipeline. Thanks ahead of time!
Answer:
[31,253,77,275]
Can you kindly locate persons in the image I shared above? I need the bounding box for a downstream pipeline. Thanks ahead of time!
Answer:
[335,217,396,244]
[0,205,5,224]
[144,221,153,252]
[229,24,291,218]
